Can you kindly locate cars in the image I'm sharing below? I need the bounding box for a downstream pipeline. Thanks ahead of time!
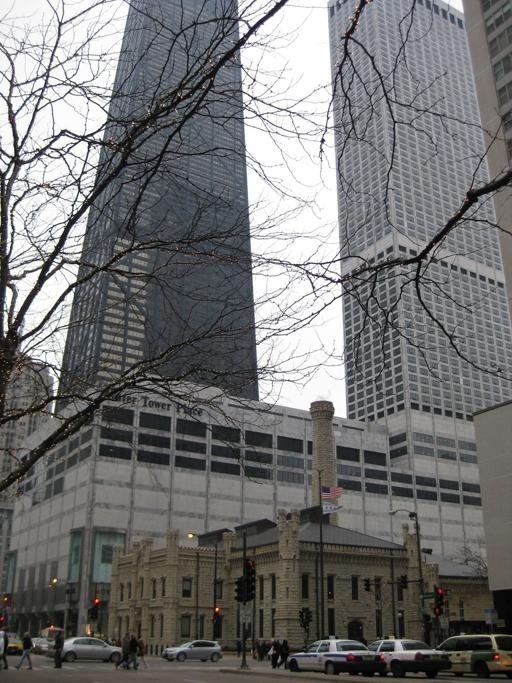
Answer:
[47,636,124,663]
[4,633,55,656]
[286,631,512,680]
[161,639,224,662]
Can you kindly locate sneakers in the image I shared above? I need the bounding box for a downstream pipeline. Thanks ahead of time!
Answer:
[3,666,9,669]
[13,665,33,670]
[272,665,288,670]
[114,664,149,671]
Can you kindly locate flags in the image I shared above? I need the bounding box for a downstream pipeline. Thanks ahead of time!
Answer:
[321,483,344,500]
[321,499,344,516]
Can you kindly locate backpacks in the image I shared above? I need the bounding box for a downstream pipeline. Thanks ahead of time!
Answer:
[267,646,274,656]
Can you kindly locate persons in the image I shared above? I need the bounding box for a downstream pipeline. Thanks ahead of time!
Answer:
[253,637,291,670]
[52,630,64,668]
[136,632,149,669]
[115,631,130,669]
[0,625,10,669]
[122,633,143,670]
[13,631,34,670]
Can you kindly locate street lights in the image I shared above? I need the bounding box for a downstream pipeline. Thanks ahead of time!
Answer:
[387,508,431,647]
[187,532,218,641]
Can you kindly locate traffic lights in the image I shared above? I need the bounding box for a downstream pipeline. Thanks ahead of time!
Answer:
[0,615,5,622]
[434,584,445,616]
[298,609,313,628]
[215,607,220,613]
[245,559,256,601]
[399,574,408,589]
[234,576,244,603]
[362,577,370,592]
[94,598,101,606]
[2,596,9,603]
